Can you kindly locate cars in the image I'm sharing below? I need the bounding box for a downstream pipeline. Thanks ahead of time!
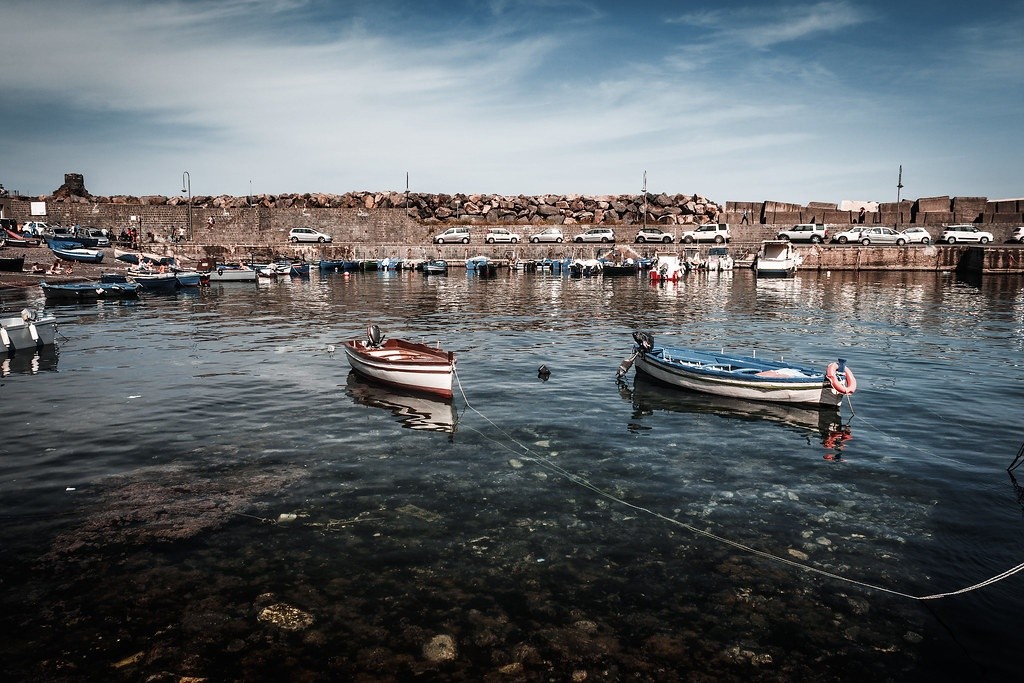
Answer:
[434,227,470,244]
[635,228,675,243]
[450,234,453,234]
[486,227,616,244]
[832,225,931,246]
[77,228,111,247]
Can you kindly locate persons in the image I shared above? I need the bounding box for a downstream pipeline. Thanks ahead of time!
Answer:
[102,227,113,241]
[51,262,73,275]
[239,259,244,268]
[738,208,748,225]
[139,259,164,274]
[120,227,137,249]
[681,250,686,262]
[267,261,276,270]
[170,225,188,243]
[31,262,43,273]
[31,220,36,235]
[67,223,81,238]
[176,258,181,268]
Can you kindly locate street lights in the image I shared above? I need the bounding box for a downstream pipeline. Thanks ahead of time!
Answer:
[641,170,647,232]
[404,172,411,236]
[181,171,193,242]
[896,165,904,231]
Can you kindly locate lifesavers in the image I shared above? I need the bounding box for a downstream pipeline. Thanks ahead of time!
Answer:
[218,269,223,276]
[826,362,857,395]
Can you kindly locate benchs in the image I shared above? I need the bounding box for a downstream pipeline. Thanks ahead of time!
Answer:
[733,368,761,372]
[379,354,421,357]
[702,364,731,367]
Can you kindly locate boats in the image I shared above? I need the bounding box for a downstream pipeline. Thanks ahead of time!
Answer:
[615,330,848,406]
[320,240,799,279]
[343,325,457,398]
[0,309,58,354]
[631,372,854,462]
[0,228,310,300]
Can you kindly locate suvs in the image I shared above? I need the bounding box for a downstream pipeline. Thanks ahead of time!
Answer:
[681,224,732,243]
[778,224,828,244]
[288,228,333,243]
[940,225,994,245]
[1010,226,1024,243]
[0,218,74,241]
